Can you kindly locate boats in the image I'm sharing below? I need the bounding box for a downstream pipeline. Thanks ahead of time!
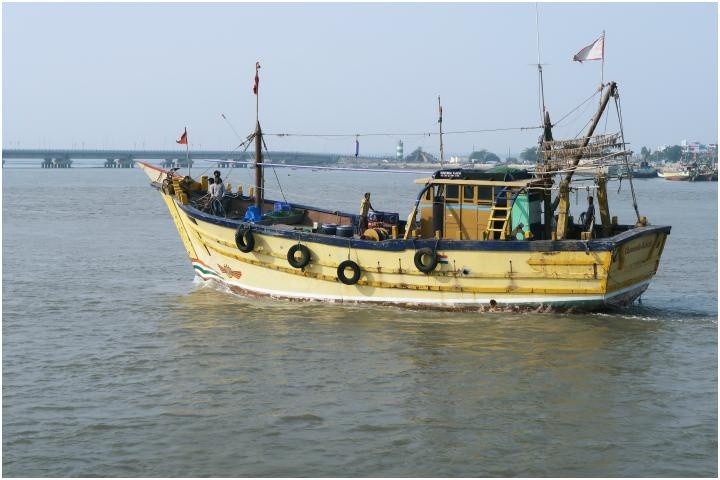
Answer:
[132,60,717,311]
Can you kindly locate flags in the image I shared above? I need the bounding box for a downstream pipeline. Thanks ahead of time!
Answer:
[571,36,604,63]
[176,131,188,145]
[252,72,260,95]
[578,196,596,231]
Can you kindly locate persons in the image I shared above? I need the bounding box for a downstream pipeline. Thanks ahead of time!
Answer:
[210,177,226,217]
[357,191,378,239]
[213,170,223,184]
[207,178,215,203]
